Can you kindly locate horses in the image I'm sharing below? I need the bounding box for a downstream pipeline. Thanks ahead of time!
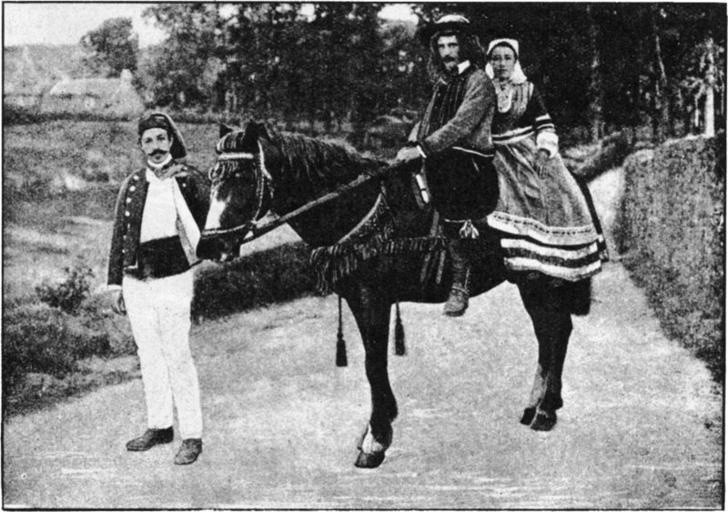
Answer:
[196,117,594,471]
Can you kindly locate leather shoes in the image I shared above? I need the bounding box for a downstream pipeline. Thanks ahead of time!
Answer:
[126,426,173,451]
[174,438,202,465]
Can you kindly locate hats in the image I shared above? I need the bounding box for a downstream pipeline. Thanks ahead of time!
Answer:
[435,15,472,35]
[138,112,188,159]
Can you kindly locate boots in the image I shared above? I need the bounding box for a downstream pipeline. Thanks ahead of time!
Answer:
[443,240,473,315]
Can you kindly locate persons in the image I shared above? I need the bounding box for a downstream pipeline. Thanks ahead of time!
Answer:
[482,38,602,288]
[106,111,211,466]
[394,13,496,316]
